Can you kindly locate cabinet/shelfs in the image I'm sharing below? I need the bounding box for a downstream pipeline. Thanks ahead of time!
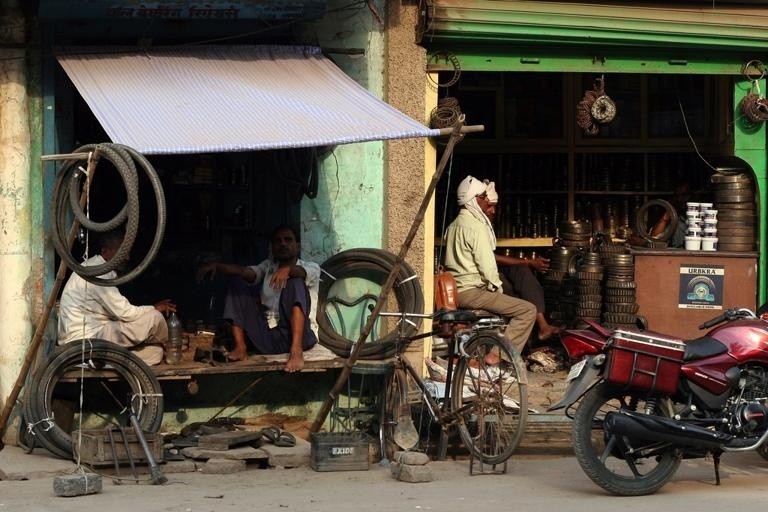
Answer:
[433,77,730,248]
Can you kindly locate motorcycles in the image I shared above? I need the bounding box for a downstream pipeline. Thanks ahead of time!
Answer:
[544,299,768,499]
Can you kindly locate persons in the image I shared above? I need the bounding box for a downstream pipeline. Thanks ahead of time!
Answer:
[494,254,567,342]
[647,178,701,250]
[55,236,178,366]
[446,174,536,370]
[206,226,321,372]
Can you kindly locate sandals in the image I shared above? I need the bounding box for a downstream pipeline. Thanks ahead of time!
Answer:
[261,426,296,447]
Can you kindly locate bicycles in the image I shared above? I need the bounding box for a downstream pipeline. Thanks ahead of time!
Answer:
[366,302,532,477]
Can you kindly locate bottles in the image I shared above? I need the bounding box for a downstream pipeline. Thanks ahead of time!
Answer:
[165,311,183,364]
